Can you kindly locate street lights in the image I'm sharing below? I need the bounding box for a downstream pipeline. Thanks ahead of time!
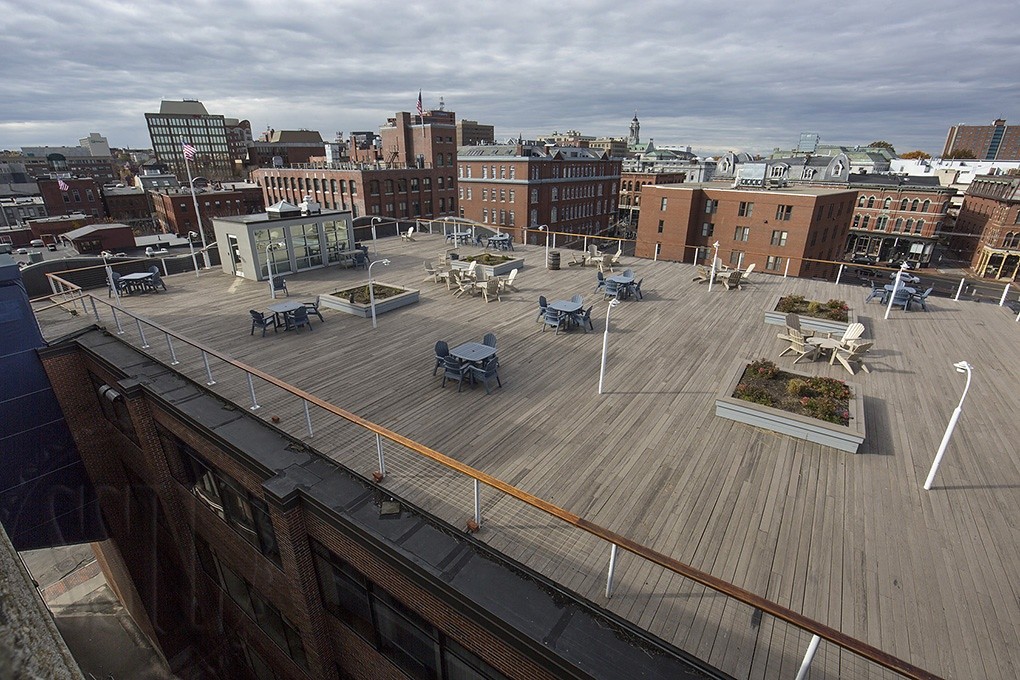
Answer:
[186,230,200,276]
[598,297,620,393]
[922,360,973,491]
[368,259,391,327]
[266,242,285,298]
[445,216,458,249]
[884,262,907,318]
[100,250,124,311]
[370,216,382,257]
[538,224,550,267]
[708,241,720,292]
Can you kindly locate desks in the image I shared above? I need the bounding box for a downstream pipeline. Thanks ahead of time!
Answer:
[266,301,303,333]
[339,249,366,269]
[806,336,839,362]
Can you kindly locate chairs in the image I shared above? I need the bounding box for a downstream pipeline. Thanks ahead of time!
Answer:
[400,226,414,246]
[779,326,816,365]
[288,306,313,335]
[828,323,865,352]
[249,310,278,338]
[691,257,755,291]
[303,295,324,322]
[865,278,933,312]
[446,227,515,251]
[785,314,814,342]
[432,332,504,396]
[106,265,167,294]
[831,337,872,375]
[273,276,289,298]
[421,253,519,304]
[534,293,595,335]
[587,244,643,301]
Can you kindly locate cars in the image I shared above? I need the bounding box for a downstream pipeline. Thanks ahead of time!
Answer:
[855,267,882,276]
[850,252,874,265]
[887,259,915,270]
[18,248,27,254]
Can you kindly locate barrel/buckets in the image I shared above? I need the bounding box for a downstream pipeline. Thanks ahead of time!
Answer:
[547,251,561,270]
